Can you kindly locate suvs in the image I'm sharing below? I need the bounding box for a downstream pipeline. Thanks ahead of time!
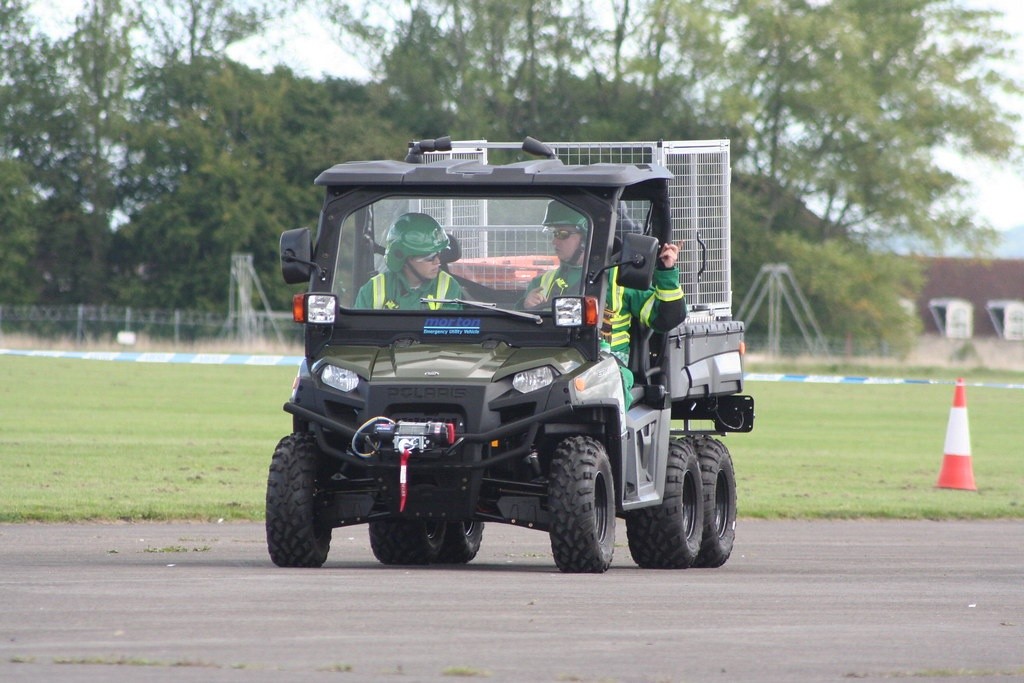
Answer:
[265,137,755,572]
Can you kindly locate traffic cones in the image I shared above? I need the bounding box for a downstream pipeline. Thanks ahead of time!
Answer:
[936,374,977,494]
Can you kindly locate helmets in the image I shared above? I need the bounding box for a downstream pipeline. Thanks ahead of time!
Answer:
[383,212,449,272]
[543,201,591,238]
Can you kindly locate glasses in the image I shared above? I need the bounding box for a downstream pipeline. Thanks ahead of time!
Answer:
[551,230,580,238]
[407,250,442,263]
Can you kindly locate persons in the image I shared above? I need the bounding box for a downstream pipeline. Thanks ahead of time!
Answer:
[516,201,687,411]
[354,212,463,310]
